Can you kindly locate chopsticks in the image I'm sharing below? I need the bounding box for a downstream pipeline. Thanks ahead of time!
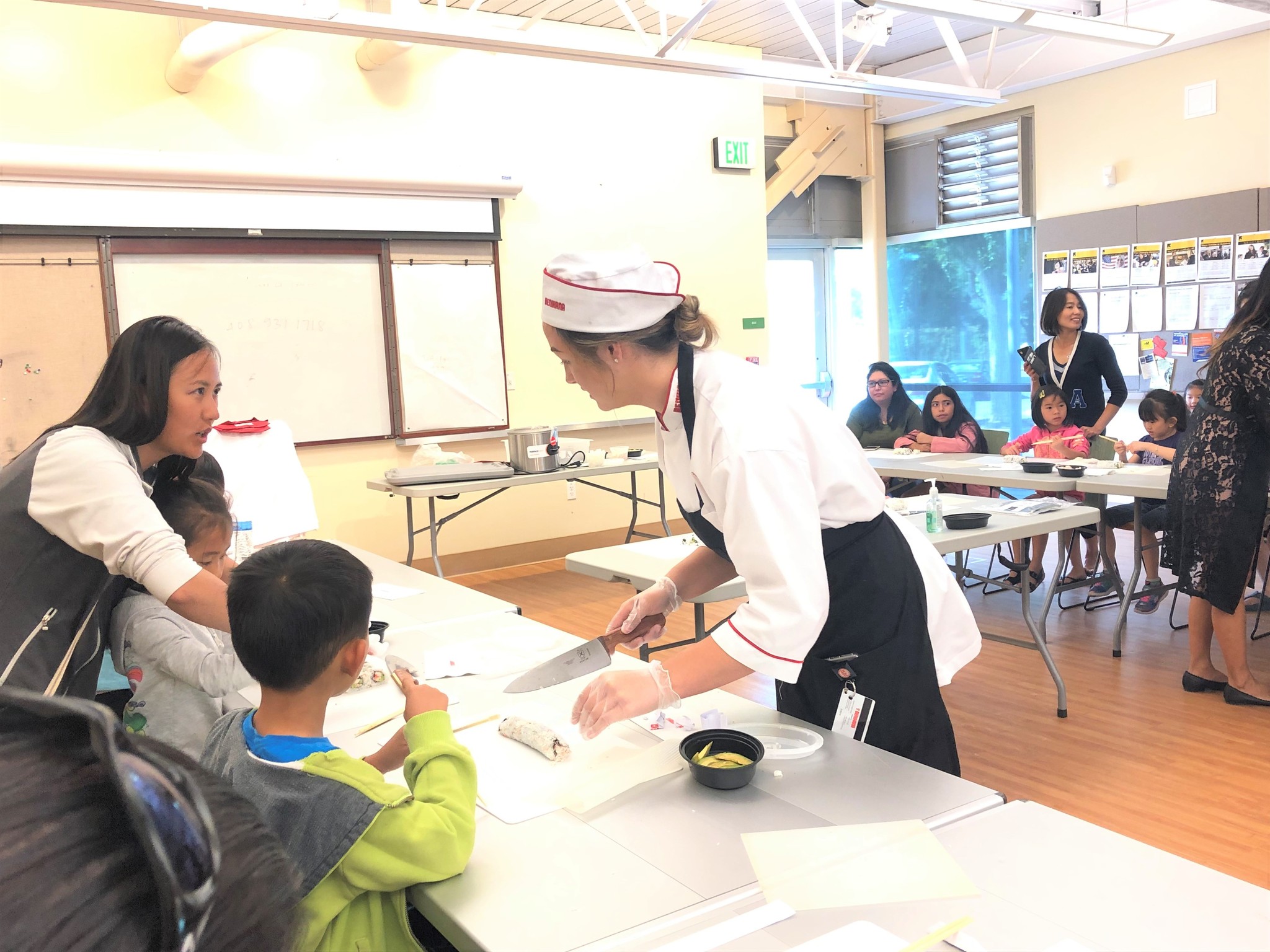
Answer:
[1031,434,1083,445]
[1098,435,1127,448]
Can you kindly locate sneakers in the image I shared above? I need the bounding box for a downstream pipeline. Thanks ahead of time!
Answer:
[1088,569,1125,596]
[1134,579,1169,614]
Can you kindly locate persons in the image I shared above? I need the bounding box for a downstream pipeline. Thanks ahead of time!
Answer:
[1052,245,1269,274]
[542,254,984,777]
[845,361,924,499]
[893,385,1001,501]
[999,385,1090,593]
[106,449,261,765]
[0,316,223,716]
[1088,388,1192,614]
[1159,257,1270,707]
[196,539,477,951]
[1023,287,1128,588]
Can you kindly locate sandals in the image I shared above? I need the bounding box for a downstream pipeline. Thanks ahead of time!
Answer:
[1015,566,1045,594]
[1001,564,1031,589]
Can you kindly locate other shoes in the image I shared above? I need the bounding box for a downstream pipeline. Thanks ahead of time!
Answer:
[1223,678,1270,707]
[1182,670,1229,693]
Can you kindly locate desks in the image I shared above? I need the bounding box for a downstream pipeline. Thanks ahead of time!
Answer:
[219,447,1270,952]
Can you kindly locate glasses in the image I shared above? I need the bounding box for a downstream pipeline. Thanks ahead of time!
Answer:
[866,379,896,388]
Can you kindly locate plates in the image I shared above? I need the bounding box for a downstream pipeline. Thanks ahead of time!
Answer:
[728,724,824,760]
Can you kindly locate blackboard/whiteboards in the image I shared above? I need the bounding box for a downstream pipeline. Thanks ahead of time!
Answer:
[388,258,510,439]
[99,238,396,446]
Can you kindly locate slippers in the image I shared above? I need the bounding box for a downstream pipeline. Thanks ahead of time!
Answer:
[1057,573,1086,587]
[1244,591,1270,613]
[1084,557,1101,576]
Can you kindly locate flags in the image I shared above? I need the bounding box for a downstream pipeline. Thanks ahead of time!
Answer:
[1102,255,1128,269]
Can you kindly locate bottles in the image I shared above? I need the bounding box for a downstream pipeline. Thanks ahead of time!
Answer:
[228,499,254,561]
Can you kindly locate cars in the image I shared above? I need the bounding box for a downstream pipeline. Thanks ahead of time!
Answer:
[890,359,990,413]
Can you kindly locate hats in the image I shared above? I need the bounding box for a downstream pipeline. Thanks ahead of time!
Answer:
[541,247,686,333]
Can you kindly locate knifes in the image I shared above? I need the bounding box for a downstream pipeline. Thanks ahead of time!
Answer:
[502,612,666,694]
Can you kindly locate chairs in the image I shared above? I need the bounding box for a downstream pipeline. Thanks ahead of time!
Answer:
[0,688,222,951]
[963,429,1190,628]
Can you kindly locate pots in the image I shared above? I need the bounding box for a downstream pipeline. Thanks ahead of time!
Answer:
[507,425,560,472]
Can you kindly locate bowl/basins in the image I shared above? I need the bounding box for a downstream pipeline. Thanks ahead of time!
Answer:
[1056,465,1087,477]
[628,449,643,457]
[679,728,765,789]
[1020,462,1055,473]
[943,513,992,529]
[585,452,606,467]
[610,446,629,459]
